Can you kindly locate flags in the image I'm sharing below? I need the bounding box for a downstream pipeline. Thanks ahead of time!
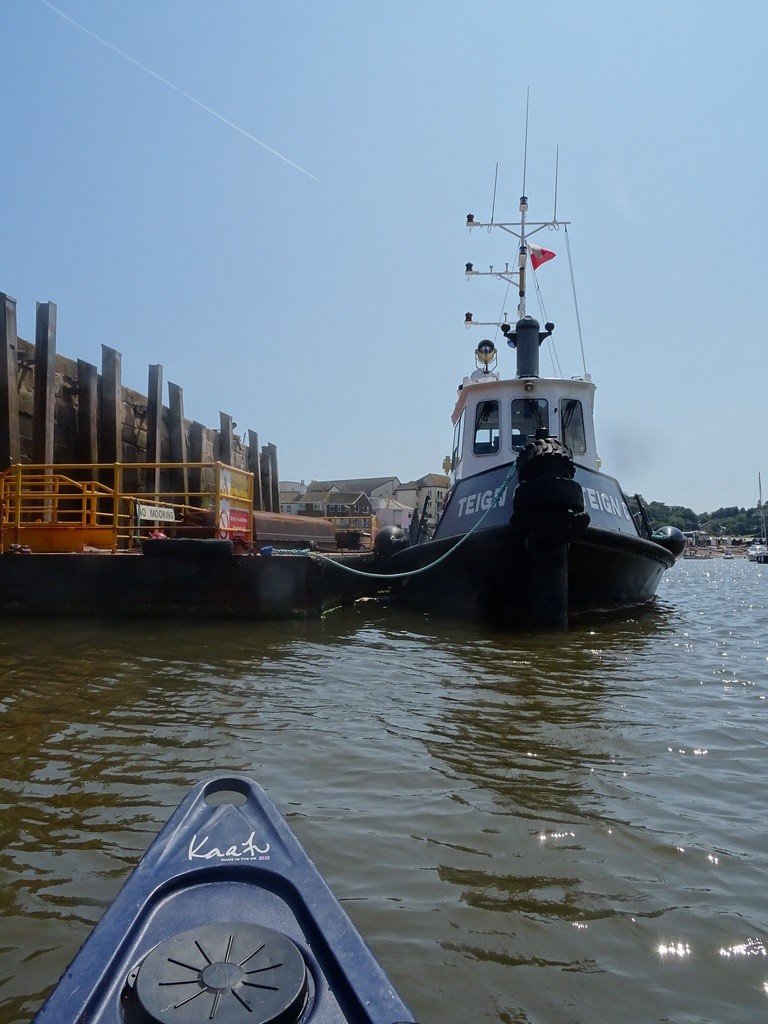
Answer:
[528,243,557,270]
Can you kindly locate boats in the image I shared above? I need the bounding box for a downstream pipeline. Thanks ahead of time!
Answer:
[748,545,767,562]
[375,87,687,632]
